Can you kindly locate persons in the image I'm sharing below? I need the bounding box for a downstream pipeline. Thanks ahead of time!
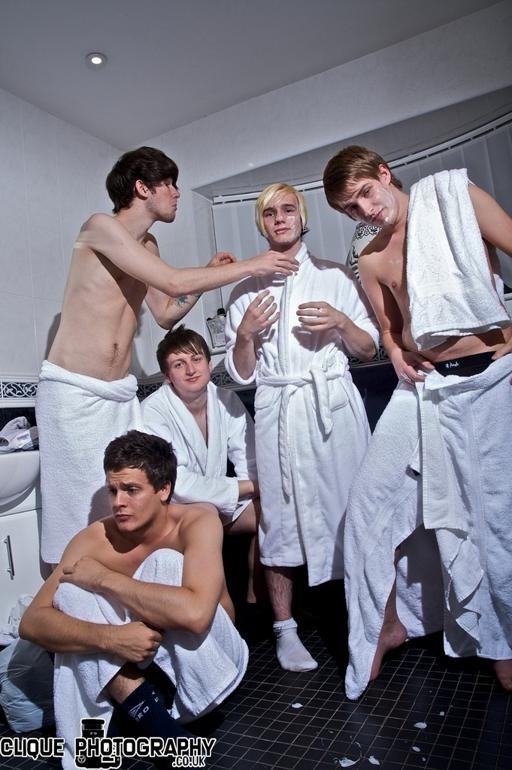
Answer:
[323,146,512,692]
[225,182,380,673]
[35,146,299,572]
[18,430,235,770]
[141,323,268,604]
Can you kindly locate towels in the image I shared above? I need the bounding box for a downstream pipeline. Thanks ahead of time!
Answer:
[342,166,512,702]
[33,358,249,770]
